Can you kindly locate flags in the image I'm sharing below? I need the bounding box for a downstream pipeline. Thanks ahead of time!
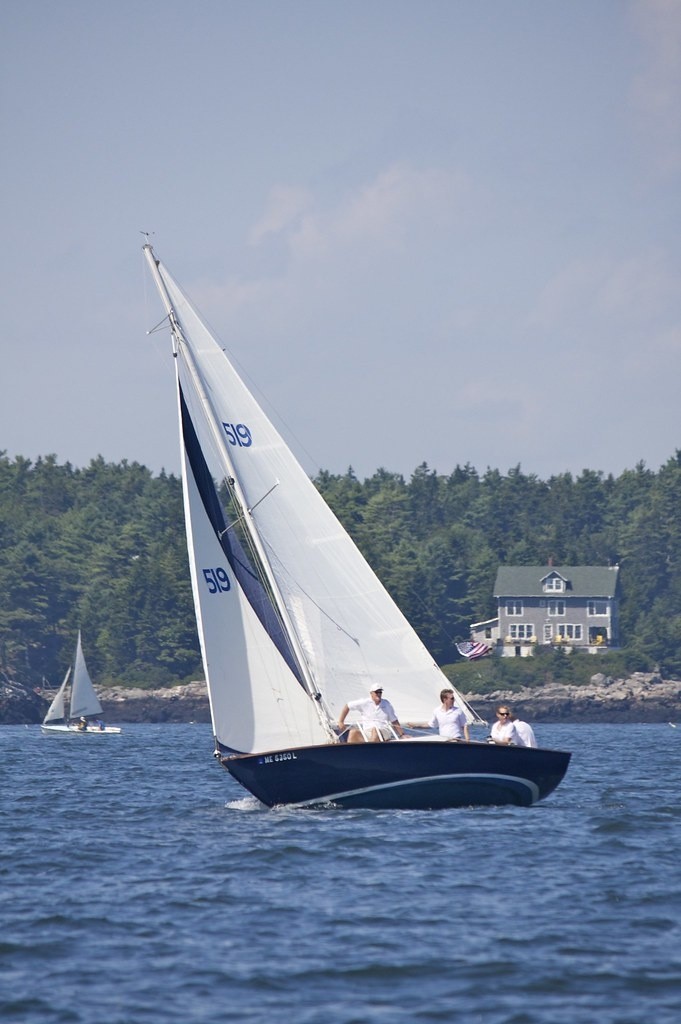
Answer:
[454,641,492,660]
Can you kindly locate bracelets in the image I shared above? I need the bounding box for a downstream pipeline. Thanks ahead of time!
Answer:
[399,733,404,737]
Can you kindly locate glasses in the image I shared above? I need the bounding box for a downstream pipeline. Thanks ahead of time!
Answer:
[447,697,455,700]
[498,713,510,716]
[374,691,383,694]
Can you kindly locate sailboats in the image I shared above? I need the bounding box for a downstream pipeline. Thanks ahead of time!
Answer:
[139,224,574,810]
[38,627,123,733]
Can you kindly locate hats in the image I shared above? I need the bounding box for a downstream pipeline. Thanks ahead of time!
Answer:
[370,684,383,691]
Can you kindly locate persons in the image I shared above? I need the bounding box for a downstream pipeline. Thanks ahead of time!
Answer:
[486,705,538,748]
[76,716,87,731]
[404,688,470,744]
[95,719,105,730]
[338,683,412,744]
